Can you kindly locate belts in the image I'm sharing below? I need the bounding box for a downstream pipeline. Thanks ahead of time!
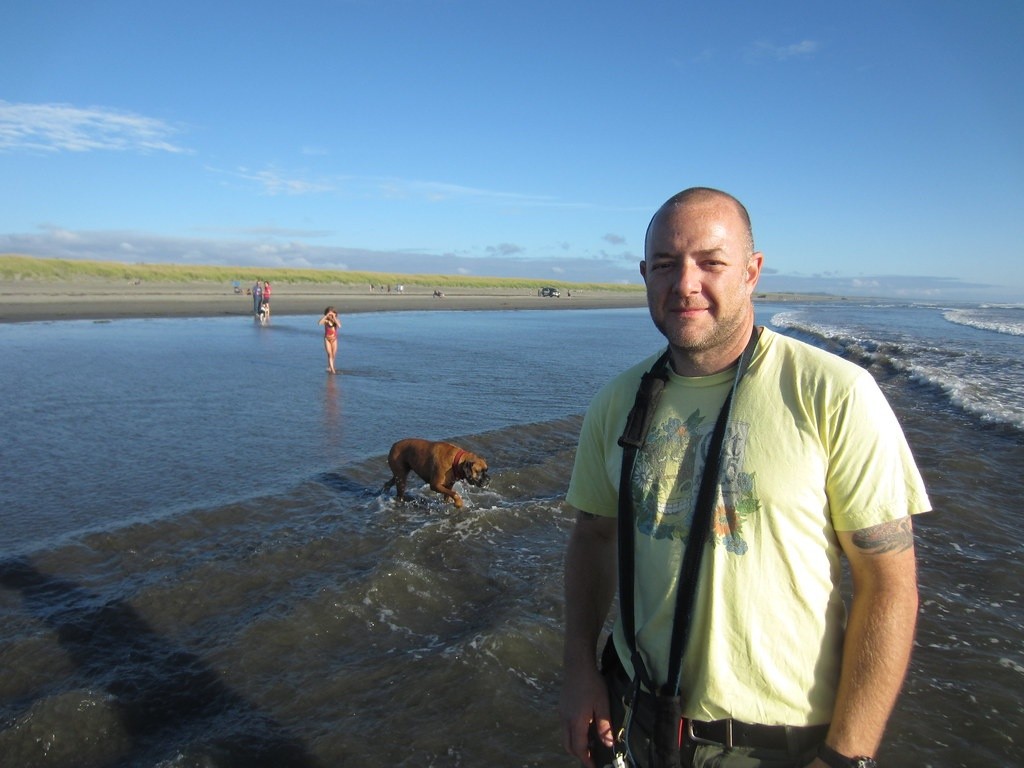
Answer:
[602,632,831,754]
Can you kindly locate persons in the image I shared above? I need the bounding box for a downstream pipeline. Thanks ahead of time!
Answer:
[253,280,272,322]
[568,290,571,300]
[319,306,341,373]
[433,290,446,298]
[369,283,405,294]
[565,187,933,768]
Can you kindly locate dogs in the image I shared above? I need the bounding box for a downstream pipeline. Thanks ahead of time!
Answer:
[374,437,491,510]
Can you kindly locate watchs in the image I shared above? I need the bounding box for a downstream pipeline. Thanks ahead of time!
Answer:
[817,742,876,768]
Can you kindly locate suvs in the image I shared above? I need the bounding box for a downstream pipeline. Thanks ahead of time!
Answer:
[542,288,560,297]
